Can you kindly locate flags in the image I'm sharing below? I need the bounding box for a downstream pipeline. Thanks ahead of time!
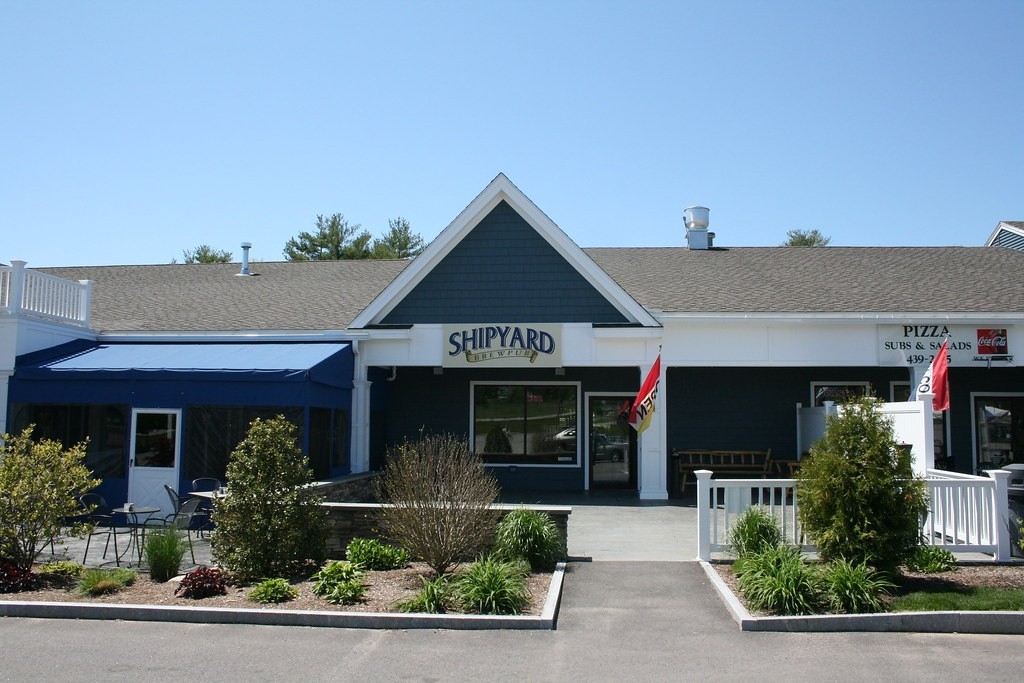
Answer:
[909,341,949,411]
[627,356,660,432]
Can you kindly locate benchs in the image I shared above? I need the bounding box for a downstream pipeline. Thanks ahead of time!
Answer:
[672,449,773,496]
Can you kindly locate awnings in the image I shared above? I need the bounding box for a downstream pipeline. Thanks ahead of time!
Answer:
[13,343,355,407]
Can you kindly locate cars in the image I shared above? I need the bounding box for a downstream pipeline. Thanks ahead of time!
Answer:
[540,426,624,462]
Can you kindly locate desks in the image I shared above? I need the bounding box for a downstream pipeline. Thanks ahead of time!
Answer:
[188,492,227,539]
[113,508,161,562]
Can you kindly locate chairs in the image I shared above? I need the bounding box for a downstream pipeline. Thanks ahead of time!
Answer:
[774,452,810,498]
[79,477,221,569]
